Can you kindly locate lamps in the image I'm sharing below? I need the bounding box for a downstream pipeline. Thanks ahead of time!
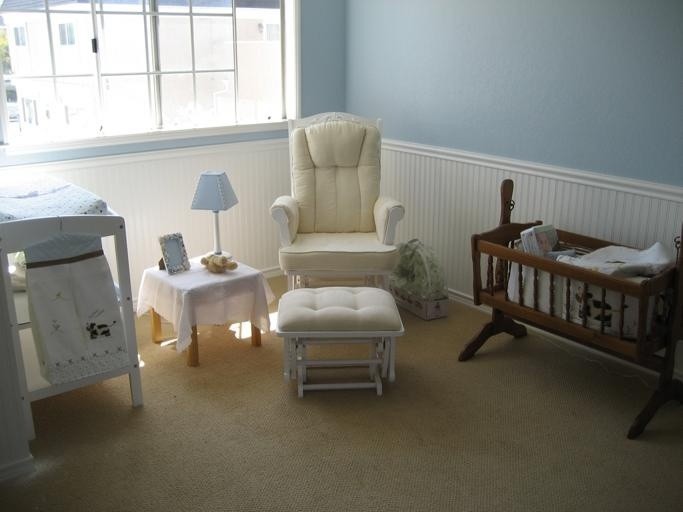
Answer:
[190,170,239,261]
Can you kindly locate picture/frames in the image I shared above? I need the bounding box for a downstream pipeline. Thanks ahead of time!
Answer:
[159,232,191,275]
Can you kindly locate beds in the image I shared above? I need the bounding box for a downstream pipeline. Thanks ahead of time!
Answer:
[0,182,143,440]
[458,179,683,439]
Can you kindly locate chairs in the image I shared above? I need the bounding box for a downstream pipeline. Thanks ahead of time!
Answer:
[269,111,405,290]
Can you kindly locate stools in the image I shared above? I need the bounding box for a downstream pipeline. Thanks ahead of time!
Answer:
[276,286,405,398]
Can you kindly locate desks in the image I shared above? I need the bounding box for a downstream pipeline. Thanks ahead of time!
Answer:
[136,254,276,367]
